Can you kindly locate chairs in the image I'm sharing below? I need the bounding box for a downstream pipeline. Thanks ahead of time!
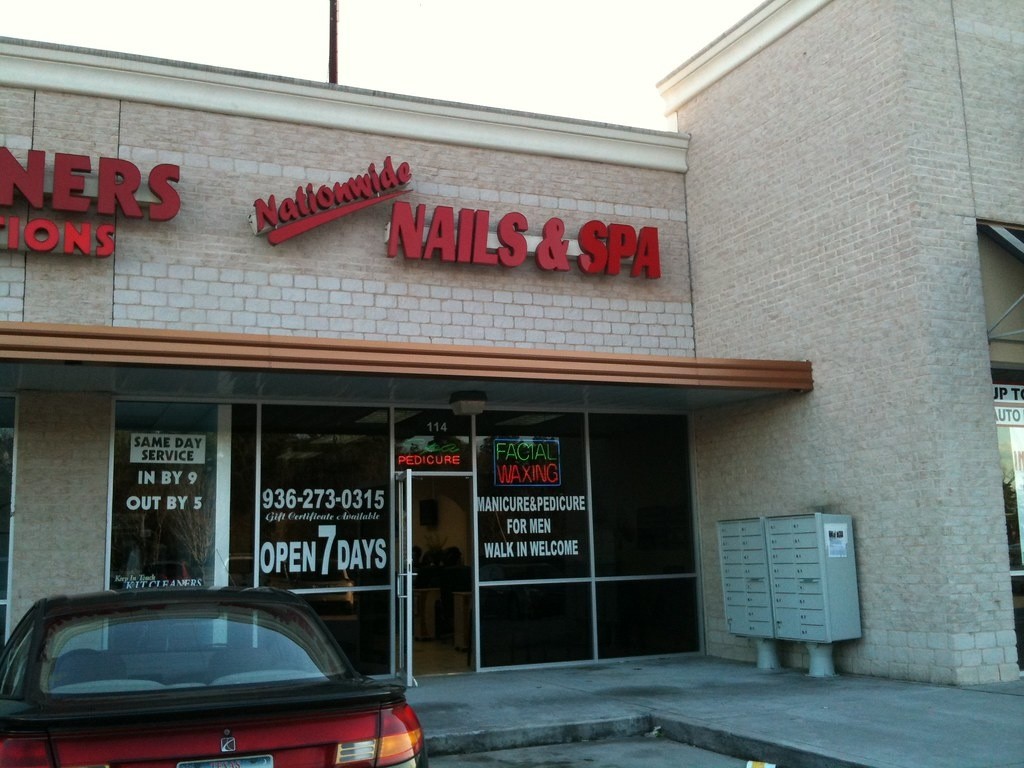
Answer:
[51,647,125,689]
[274,579,360,656]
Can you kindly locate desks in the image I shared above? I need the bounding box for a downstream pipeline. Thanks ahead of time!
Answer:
[412,588,441,641]
[453,591,473,651]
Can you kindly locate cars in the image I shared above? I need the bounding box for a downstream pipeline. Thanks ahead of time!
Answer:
[0,587,430,768]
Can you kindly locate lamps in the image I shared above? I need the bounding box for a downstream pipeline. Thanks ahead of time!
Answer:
[448,392,490,415]
